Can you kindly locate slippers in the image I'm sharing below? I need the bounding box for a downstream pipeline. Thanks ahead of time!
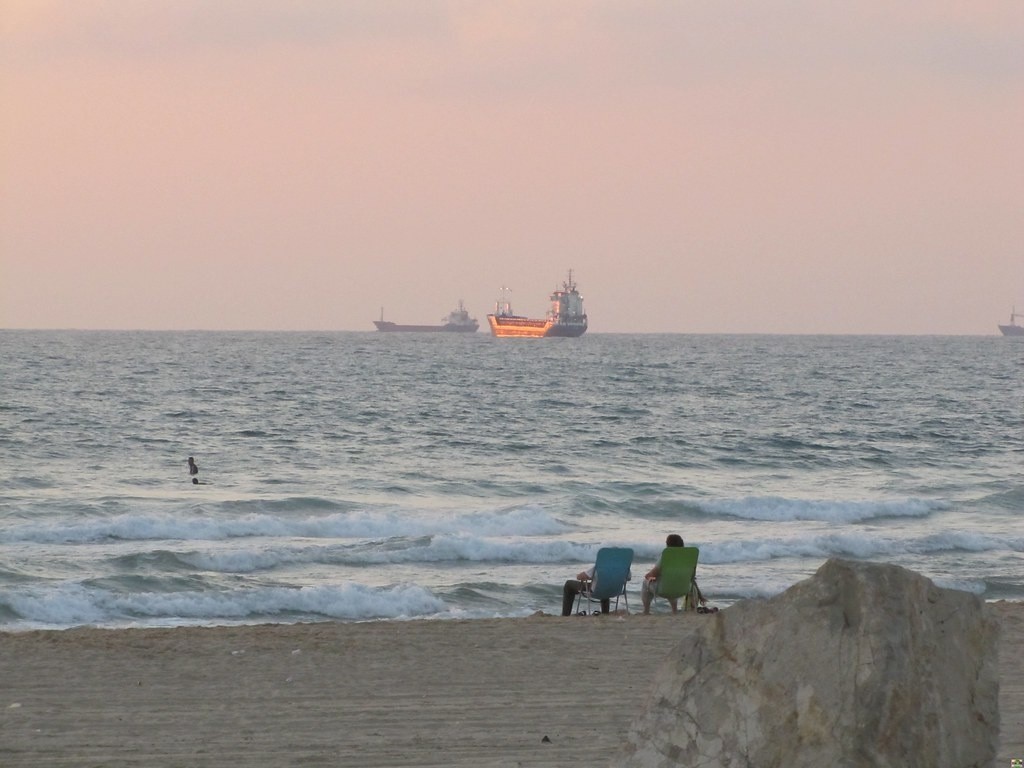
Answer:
[592,610,601,615]
[578,610,587,615]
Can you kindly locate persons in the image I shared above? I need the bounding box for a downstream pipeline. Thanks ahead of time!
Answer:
[635,535,684,617]
[187,457,198,484]
[563,548,631,617]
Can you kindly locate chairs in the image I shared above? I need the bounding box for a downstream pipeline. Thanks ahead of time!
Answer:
[648,547,699,613]
[575,547,634,618]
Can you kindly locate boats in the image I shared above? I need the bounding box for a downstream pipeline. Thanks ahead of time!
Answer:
[996,304,1024,336]
[484,268,589,337]
[371,295,481,334]
